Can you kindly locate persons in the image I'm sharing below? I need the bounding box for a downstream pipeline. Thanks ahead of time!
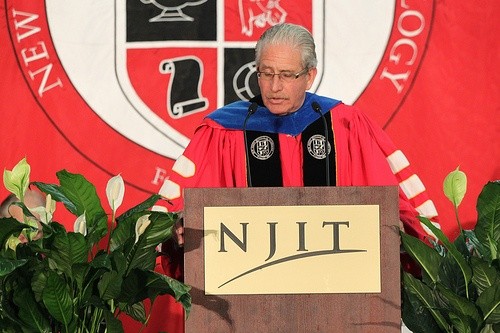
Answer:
[151,23,441,282]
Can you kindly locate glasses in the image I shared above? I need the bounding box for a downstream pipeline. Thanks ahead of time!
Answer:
[256,66,307,82]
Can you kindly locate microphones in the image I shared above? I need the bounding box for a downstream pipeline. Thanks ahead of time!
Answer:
[243,103,258,187]
[311,102,330,186]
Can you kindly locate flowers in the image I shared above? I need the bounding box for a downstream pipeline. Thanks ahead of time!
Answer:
[1,157,159,256]
[442,165,468,208]
